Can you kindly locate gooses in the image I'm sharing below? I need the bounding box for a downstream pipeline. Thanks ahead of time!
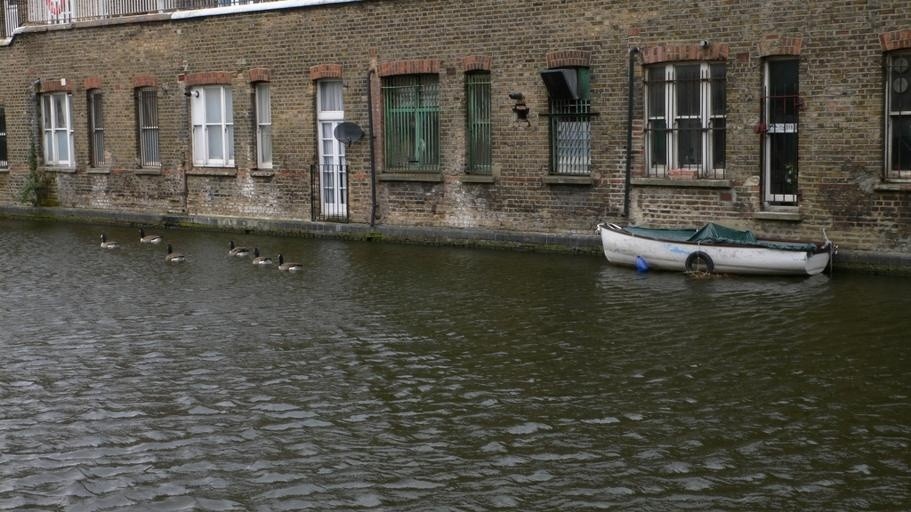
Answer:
[165,244,185,263]
[252,247,272,265]
[228,240,250,256]
[100,232,120,249]
[138,228,163,244]
[274,254,304,271]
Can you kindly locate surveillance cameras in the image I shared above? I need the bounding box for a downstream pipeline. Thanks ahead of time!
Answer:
[509,93,523,100]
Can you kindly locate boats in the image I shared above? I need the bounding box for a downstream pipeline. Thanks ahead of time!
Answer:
[596,221,832,275]
